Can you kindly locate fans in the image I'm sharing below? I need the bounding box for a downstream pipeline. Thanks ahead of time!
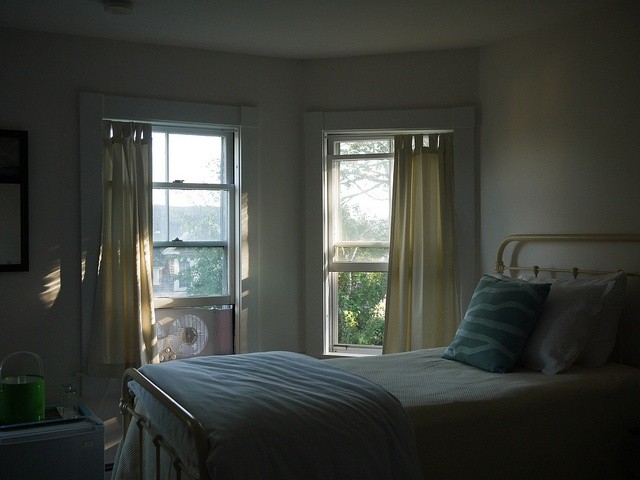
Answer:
[167,314,210,358]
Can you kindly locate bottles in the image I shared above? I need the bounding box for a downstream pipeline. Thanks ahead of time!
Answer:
[58,384,79,421]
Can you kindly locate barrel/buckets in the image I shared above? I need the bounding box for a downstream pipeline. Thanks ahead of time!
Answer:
[0,348,47,427]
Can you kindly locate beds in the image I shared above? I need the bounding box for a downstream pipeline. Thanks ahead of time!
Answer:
[111,233,639,480]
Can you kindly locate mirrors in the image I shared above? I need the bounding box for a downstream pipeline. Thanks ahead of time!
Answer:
[0,128,29,273]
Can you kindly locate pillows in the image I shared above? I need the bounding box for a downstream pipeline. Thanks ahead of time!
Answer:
[443,276,550,376]
[527,271,628,376]
